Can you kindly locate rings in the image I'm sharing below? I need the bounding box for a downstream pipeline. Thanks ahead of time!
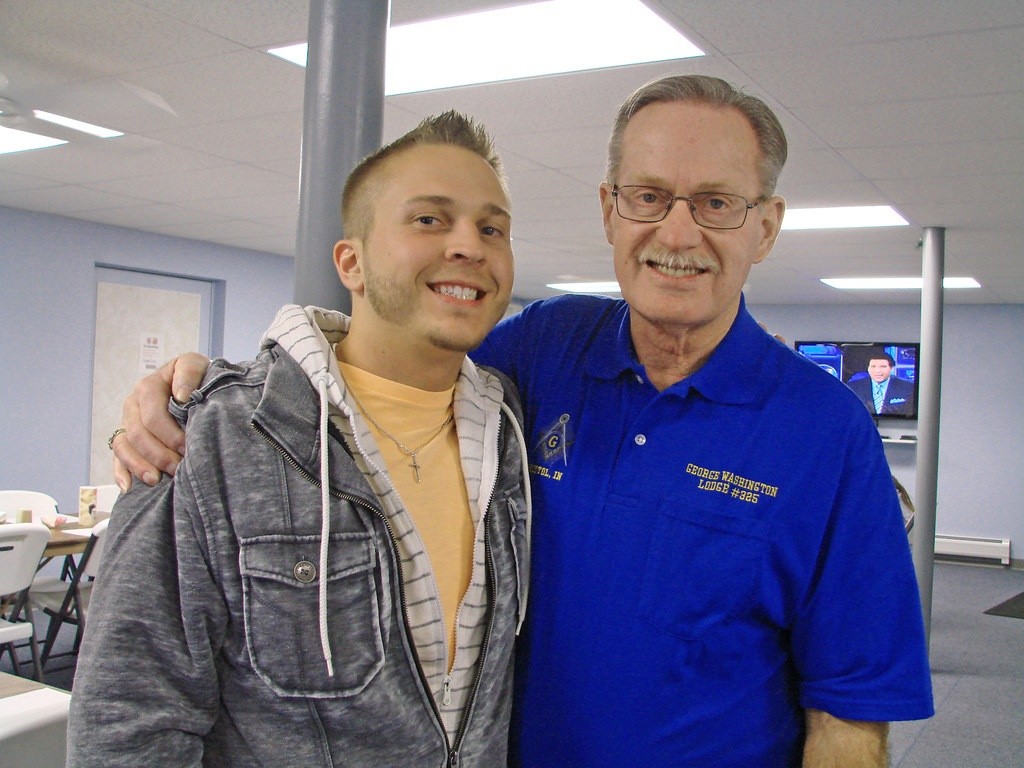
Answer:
[107,428,126,450]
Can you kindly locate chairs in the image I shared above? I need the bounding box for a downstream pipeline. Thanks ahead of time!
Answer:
[0,485,127,681]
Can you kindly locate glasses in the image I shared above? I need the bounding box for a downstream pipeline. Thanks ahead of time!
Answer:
[612,185,762,229]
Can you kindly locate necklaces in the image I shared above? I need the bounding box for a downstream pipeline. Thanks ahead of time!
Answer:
[338,368,454,483]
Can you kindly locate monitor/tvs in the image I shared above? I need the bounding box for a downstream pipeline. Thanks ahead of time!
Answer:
[795,341,921,420]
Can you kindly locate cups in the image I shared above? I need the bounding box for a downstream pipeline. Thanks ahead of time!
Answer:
[79,486,98,526]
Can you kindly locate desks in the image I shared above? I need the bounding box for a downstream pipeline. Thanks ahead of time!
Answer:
[0,511,111,680]
[0,670,73,767]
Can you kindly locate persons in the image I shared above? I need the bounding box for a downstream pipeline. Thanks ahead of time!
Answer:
[847,352,914,416]
[101,71,936,768]
[64,109,540,767]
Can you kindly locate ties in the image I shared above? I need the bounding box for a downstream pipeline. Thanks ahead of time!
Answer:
[873,385,883,413]
